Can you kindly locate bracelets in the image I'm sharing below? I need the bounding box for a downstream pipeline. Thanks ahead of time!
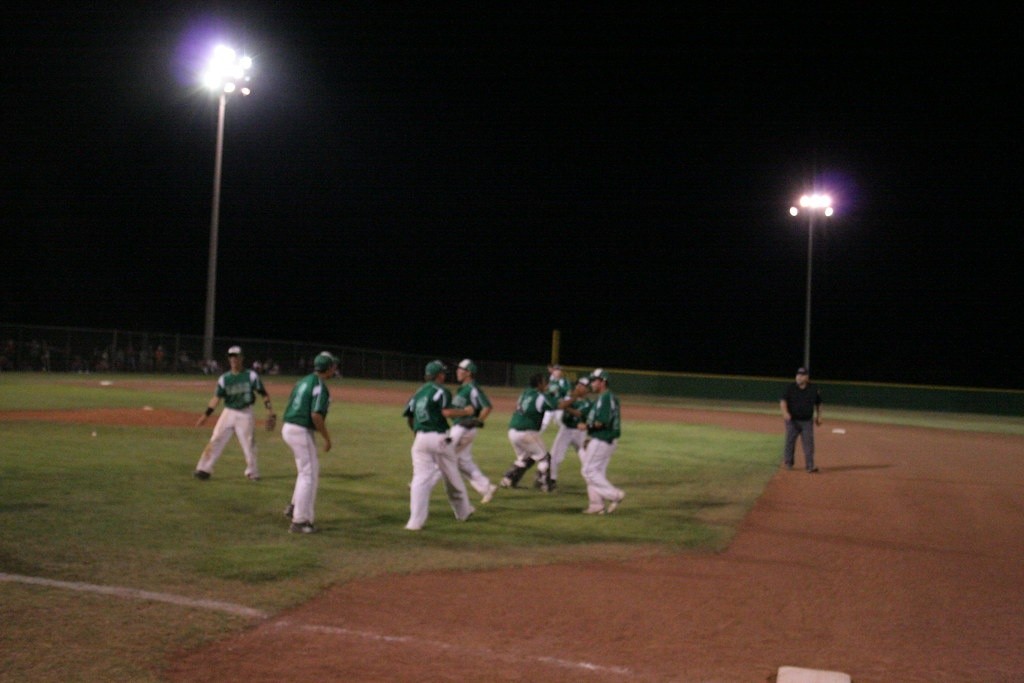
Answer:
[587,424,596,429]
[205,407,214,416]
[263,400,272,409]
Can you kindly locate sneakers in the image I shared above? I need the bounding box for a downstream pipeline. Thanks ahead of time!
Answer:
[466,510,489,522]
[500,476,511,488]
[288,521,322,535]
[195,471,210,480]
[607,488,625,514]
[583,504,606,514]
[284,505,293,518]
[248,473,260,481]
[480,484,498,503]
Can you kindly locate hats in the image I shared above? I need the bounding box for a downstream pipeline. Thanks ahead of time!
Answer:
[589,368,611,386]
[314,351,341,371]
[577,376,592,392]
[798,367,808,374]
[454,359,478,376]
[225,345,244,356]
[425,360,451,376]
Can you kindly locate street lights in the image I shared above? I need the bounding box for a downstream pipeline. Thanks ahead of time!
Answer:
[200,48,248,360]
[798,192,830,376]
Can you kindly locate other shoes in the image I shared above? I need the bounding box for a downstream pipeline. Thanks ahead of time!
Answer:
[786,463,791,469]
[806,465,818,472]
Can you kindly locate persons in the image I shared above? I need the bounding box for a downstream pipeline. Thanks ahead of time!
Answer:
[500,363,598,491]
[280,350,338,534]
[0,338,343,378]
[193,345,277,482]
[402,359,476,531]
[577,367,626,515]
[780,367,824,474]
[408,360,498,507]
